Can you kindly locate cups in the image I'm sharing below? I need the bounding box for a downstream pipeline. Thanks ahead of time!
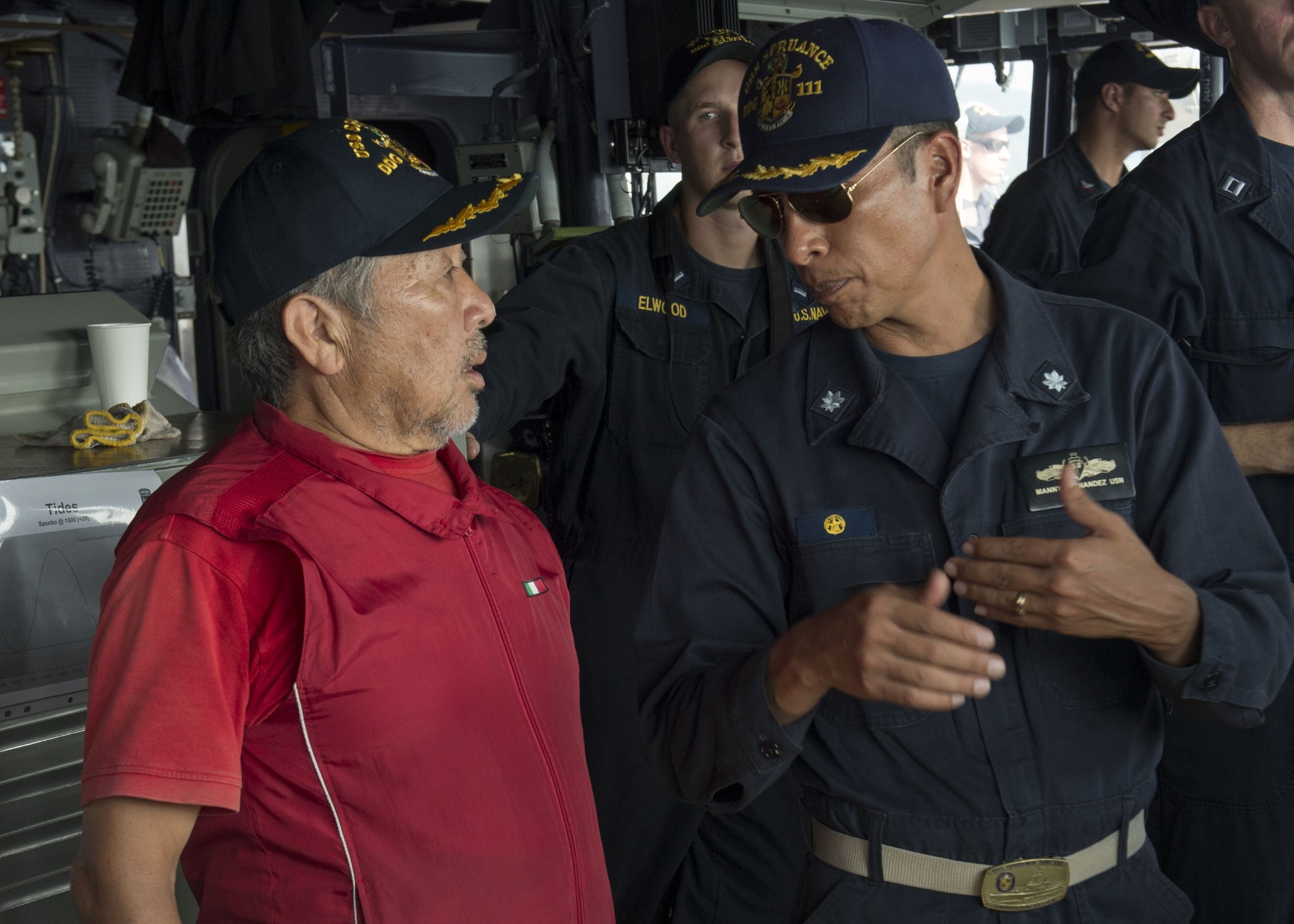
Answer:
[86,323,152,410]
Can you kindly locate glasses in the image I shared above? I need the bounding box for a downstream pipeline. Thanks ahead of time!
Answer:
[736,133,931,241]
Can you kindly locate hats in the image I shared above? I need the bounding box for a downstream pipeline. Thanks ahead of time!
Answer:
[1079,39,1203,99]
[661,28,761,124]
[694,16,959,217]
[211,117,538,327]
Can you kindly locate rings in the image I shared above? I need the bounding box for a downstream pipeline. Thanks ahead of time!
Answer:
[1015,591,1027,617]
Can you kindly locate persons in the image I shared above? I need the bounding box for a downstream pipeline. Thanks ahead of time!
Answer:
[472,0,1293,924]
[71,114,624,924]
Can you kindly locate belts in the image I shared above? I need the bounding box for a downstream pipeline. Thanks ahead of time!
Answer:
[809,808,1149,911]
